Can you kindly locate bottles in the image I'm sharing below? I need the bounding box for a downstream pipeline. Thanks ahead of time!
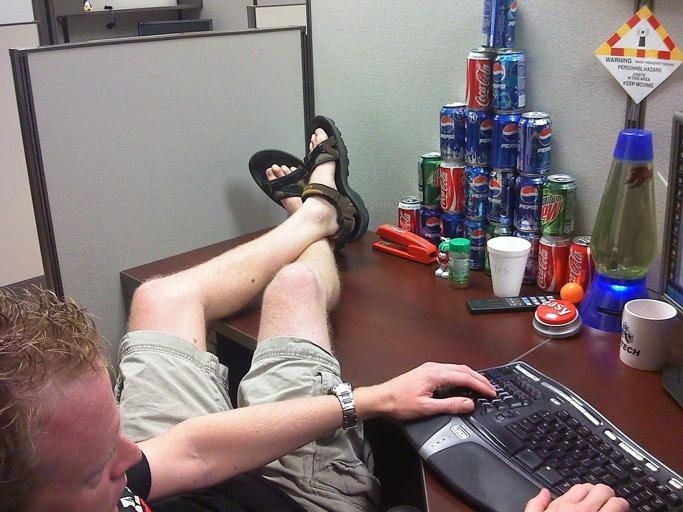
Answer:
[490,111,521,173]
[540,172,577,236]
[511,228,539,285]
[463,108,491,168]
[513,173,545,233]
[439,160,466,215]
[464,49,495,112]
[417,204,441,247]
[439,211,463,243]
[462,165,491,221]
[447,237,470,289]
[397,196,421,236]
[516,110,552,178]
[462,218,486,270]
[491,49,526,114]
[480,0,518,51]
[483,220,513,276]
[438,101,465,162]
[486,170,518,228]
[565,235,596,293]
[577,129,664,339]
[415,151,442,209]
[535,235,571,293]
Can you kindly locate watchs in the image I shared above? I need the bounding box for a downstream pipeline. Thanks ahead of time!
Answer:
[328,383,361,430]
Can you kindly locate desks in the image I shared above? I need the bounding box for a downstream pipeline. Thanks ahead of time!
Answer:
[120,199,683,512]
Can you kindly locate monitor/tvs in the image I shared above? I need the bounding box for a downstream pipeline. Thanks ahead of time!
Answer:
[137,18,211,36]
[659,109,683,410]
[61,7,182,44]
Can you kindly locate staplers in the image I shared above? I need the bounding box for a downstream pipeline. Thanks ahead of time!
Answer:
[372,224,437,264]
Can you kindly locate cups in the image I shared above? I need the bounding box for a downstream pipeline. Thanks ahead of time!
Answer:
[487,235,532,298]
[618,298,678,371]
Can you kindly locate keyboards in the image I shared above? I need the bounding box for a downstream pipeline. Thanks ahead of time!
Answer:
[396,362,683,512]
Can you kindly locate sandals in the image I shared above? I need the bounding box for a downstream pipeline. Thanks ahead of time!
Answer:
[301,118,369,243]
[249,151,349,248]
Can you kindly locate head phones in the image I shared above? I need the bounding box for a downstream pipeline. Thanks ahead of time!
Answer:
[103,6,113,30]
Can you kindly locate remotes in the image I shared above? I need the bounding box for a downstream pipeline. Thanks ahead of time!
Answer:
[466,295,557,315]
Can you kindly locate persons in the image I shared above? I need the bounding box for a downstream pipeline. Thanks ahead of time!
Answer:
[0,115,497,512]
